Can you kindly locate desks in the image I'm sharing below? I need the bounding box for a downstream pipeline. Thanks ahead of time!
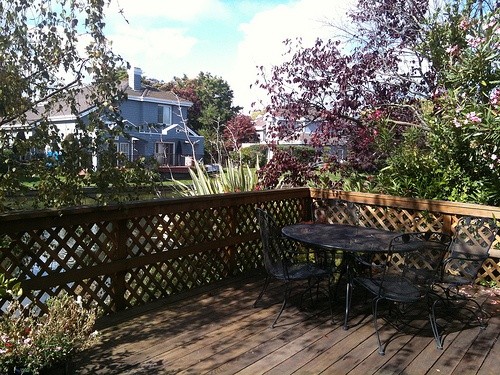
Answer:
[279,218,429,303]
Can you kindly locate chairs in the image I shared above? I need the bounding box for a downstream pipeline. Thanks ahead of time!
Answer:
[252,208,336,334]
[400,216,497,329]
[342,225,452,355]
[305,199,362,294]
